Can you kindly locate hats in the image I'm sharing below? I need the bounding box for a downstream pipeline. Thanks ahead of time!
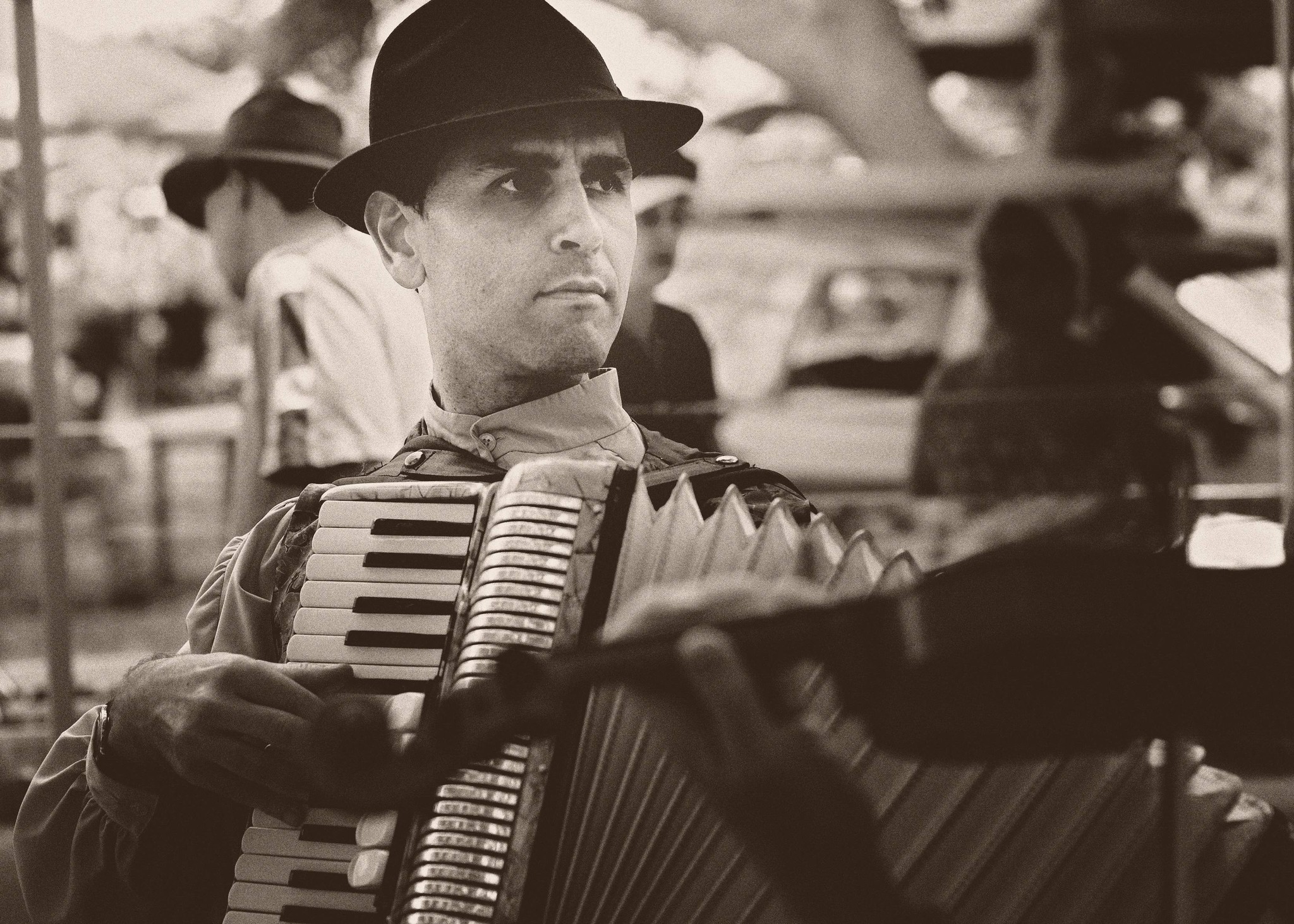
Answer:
[312,0,705,239]
[631,149,696,218]
[161,90,344,229]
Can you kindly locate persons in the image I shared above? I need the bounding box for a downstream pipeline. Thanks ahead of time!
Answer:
[600,150,721,453]
[13,0,821,924]
[162,82,433,490]
